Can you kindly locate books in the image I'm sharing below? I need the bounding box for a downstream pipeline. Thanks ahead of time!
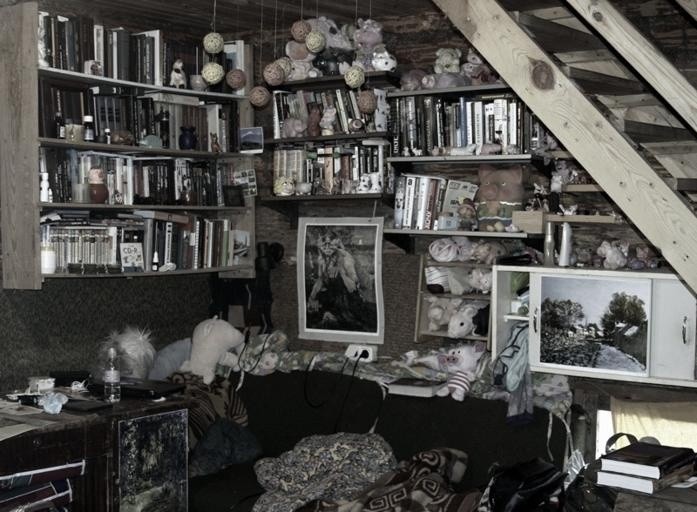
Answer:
[389,95,522,156]
[38,12,245,95]
[37,146,235,206]
[273,140,388,193]
[38,78,231,152]
[39,210,234,275]
[273,90,388,139]
[596,441,697,498]
[393,175,478,230]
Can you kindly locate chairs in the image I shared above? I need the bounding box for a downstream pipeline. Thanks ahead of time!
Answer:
[288,367,385,436]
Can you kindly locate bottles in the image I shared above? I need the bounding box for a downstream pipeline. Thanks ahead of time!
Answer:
[54,112,65,139]
[103,347,121,402]
[542,222,573,267]
[82,115,94,141]
[104,128,112,145]
[179,124,196,150]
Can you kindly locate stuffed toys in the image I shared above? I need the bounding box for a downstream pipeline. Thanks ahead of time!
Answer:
[421,233,529,338]
[265,15,500,93]
[433,339,486,402]
[184,314,246,382]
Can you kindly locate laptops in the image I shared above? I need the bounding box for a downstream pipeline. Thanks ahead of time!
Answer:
[87,376,187,399]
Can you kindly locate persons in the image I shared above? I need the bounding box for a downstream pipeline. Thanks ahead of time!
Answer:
[304,233,367,327]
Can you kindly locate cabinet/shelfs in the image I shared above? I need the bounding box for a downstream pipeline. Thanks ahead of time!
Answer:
[1,3,696,390]
